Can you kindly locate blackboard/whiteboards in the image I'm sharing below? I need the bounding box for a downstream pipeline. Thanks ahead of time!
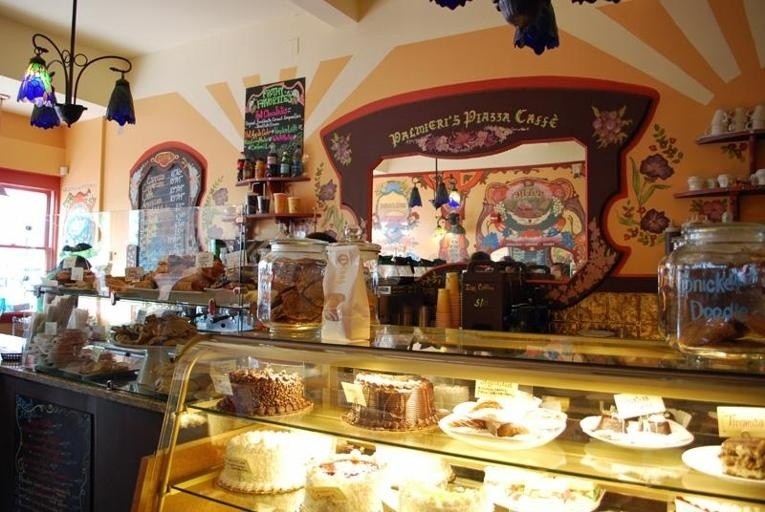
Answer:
[129,141,208,275]
[244,77,305,163]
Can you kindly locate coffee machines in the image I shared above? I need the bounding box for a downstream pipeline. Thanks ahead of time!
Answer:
[462,257,570,335]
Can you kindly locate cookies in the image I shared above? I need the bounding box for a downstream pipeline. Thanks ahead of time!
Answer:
[661,265,765,345]
[272,259,327,324]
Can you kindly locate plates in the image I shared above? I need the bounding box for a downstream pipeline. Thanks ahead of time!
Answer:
[438,394,569,447]
[565,400,696,453]
[681,443,765,488]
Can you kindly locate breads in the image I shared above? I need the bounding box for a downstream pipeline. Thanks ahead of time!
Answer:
[28,258,258,402]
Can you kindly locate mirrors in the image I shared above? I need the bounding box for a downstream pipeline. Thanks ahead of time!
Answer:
[366,135,590,287]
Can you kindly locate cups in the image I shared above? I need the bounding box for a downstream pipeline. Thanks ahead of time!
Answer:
[705,106,763,137]
[245,192,301,215]
[685,168,765,191]
[433,270,463,329]
[397,303,428,325]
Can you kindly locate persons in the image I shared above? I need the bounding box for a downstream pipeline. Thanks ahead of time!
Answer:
[36,255,91,313]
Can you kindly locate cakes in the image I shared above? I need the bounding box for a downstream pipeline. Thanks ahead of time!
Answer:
[218,428,308,493]
[348,372,440,432]
[450,387,567,447]
[300,451,384,512]
[227,367,311,416]
[395,463,494,512]
[717,436,765,479]
[595,408,670,438]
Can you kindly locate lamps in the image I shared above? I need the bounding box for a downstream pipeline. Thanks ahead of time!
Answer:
[17,0,138,135]
[405,157,463,212]
[434,213,465,239]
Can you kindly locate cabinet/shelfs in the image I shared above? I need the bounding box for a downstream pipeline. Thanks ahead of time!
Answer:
[673,127,765,200]
[231,174,325,224]
[0,274,257,512]
[139,310,765,512]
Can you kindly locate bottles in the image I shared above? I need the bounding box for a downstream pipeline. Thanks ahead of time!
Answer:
[252,225,380,336]
[654,207,764,362]
[236,143,303,181]
